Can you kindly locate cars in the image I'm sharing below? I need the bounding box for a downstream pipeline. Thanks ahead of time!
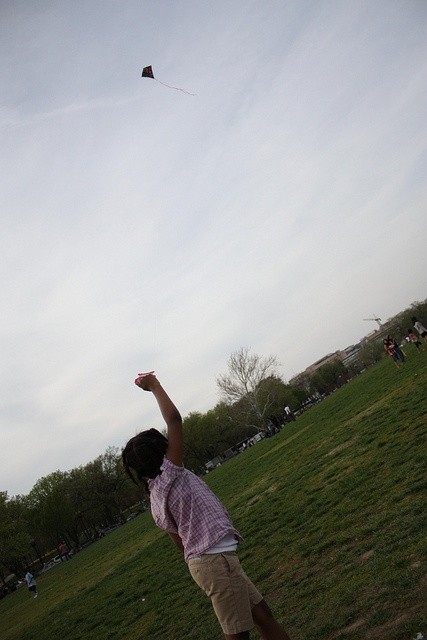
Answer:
[37,552,71,574]
[0,578,26,598]
[101,511,137,537]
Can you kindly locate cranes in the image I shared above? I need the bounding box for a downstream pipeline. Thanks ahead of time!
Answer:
[362,313,383,327]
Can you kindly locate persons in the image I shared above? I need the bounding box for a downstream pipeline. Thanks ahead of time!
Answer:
[383,339,403,369]
[386,334,404,363]
[411,316,427,343]
[408,328,424,352]
[283,404,297,422]
[266,415,283,431]
[23,569,38,599]
[405,336,410,343]
[58,542,72,561]
[121,374,292,640]
[241,439,255,449]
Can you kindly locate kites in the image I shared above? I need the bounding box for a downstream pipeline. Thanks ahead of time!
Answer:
[141,66,197,97]
